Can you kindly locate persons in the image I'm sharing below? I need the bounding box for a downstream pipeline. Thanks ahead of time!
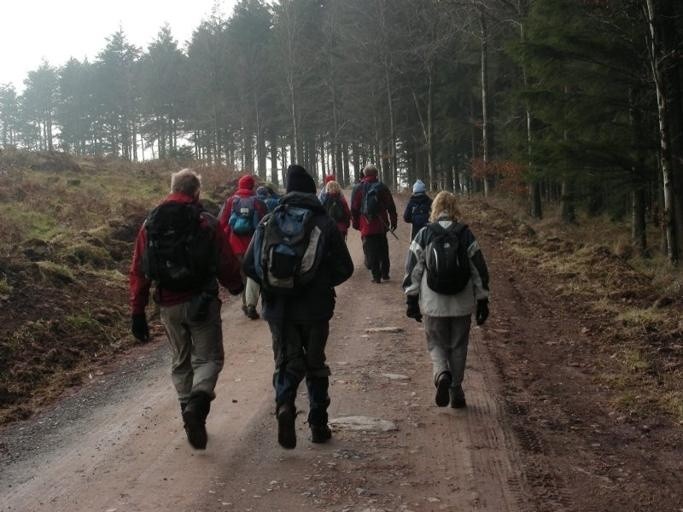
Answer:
[318,180,351,242]
[318,175,343,205]
[355,168,372,269]
[403,179,433,244]
[129,168,244,449]
[242,164,354,449]
[400,190,490,408]
[255,186,281,214]
[351,164,398,282]
[217,174,266,321]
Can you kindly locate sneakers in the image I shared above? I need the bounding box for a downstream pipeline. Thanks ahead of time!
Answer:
[435,373,450,407]
[242,305,247,314]
[248,305,259,319]
[311,424,332,444]
[184,396,210,449]
[451,397,466,408]
[277,403,296,448]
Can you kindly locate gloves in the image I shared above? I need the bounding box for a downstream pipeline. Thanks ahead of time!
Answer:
[475,299,489,325]
[406,296,422,322]
[131,315,149,342]
